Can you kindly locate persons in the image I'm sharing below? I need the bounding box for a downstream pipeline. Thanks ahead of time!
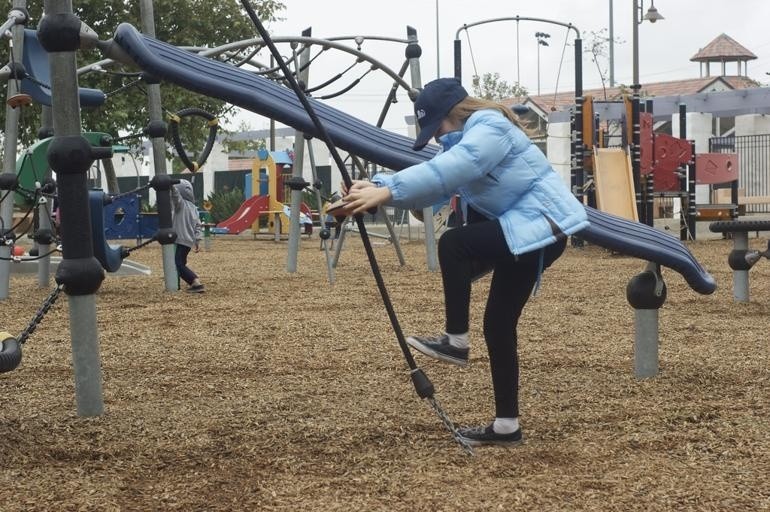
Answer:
[167,178,208,293]
[339,76,591,446]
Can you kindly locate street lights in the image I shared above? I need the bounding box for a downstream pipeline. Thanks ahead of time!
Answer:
[633,0,664,99]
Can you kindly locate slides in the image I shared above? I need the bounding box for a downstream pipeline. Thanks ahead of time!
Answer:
[592,145,640,223]
[216,195,269,234]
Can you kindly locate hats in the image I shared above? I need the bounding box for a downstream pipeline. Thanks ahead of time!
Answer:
[174,178,194,202]
[412,77,468,152]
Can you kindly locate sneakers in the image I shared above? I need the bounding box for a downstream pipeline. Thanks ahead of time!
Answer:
[404,332,471,368]
[186,284,204,295]
[455,417,523,445]
